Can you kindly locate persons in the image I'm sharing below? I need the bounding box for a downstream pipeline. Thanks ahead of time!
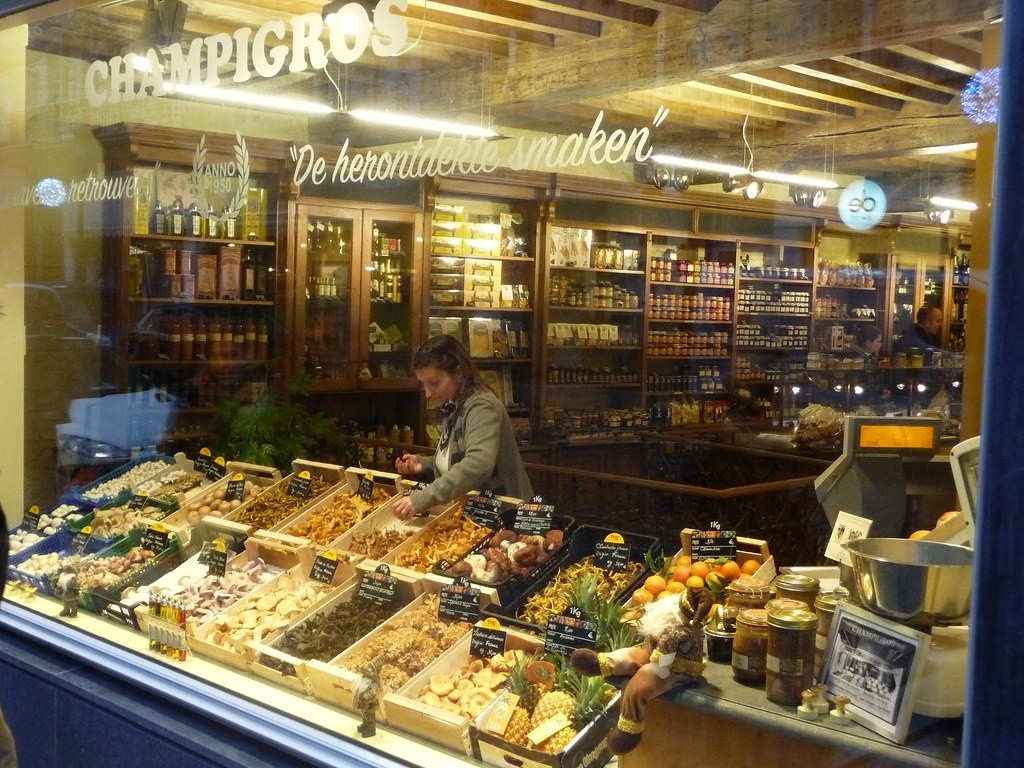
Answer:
[393,333,534,522]
[891,303,954,408]
[841,327,892,400]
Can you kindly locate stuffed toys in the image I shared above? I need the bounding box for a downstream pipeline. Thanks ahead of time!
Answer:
[569,585,717,755]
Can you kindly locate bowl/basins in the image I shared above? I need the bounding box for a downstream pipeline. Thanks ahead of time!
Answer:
[841,538,975,624]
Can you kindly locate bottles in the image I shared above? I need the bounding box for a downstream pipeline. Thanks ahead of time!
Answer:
[241,249,256,301]
[369,222,404,302]
[305,327,349,381]
[307,276,349,302]
[949,253,970,353]
[308,219,349,253]
[126,303,272,454]
[254,247,269,300]
[152,196,241,239]
[294,392,387,444]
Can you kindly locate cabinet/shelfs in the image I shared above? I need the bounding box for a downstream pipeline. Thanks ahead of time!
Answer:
[293,195,425,393]
[547,446,646,526]
[536,172,817,441]
[421,158,553,450]
[90,120,295,450]
[815,205,973,398]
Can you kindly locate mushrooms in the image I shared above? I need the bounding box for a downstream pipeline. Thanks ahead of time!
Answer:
[443,530,566,583]
[417,648,531,720]
[6,458,333,652]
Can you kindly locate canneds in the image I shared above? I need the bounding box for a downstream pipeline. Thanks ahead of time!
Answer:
[709,574,857,707]
[546,249,944,429]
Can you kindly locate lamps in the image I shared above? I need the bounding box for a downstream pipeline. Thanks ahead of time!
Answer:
[923,204,953,226]
[741,175,764,201]
[789,186,808,208]
[808,187,828,209]
[646,166,671,190]
[668,167,691,192]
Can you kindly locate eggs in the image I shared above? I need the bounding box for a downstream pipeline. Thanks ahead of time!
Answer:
[630,554,762,612]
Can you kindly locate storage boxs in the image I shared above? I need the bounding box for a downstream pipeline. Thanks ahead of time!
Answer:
[568,228,580,267]
[48,526,183,614]
[428,202,534,405]
[89,524,248,630]
[65,485,181,544]
[557,227,568,266]
[5,523,112,597]
[382,619,545,758]
[618,528,778,627]
[550,225,558,266]
[39,489,106,518]
[159,457,576,693]
[580,229,599,268]
[306,592,474,726]
[71,452,176,508]
[132,451,232,508]
[468,673,623,768]
[481,526,663,650]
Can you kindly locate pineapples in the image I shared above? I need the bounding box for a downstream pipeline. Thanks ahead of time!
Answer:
[485,571,643,754]
